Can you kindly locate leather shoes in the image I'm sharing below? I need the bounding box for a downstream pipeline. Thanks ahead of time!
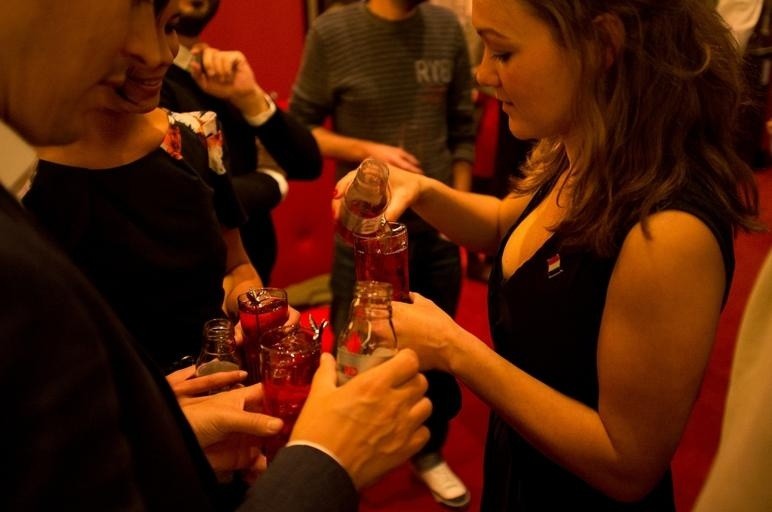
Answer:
[415,462,471,507]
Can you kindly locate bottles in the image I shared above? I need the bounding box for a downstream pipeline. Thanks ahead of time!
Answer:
[333,159,391,268]
[335,280,399,388]
[195,318,241,399]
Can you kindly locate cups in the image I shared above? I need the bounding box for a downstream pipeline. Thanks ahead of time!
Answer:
[354,221,410,305]
[236,286,322,461]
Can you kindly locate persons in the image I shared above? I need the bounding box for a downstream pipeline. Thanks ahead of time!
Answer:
[0,0,432,512]
[330,0,760,512]
[291,0,483,512]
[470,89,541,285]
[698,248,772,512]
